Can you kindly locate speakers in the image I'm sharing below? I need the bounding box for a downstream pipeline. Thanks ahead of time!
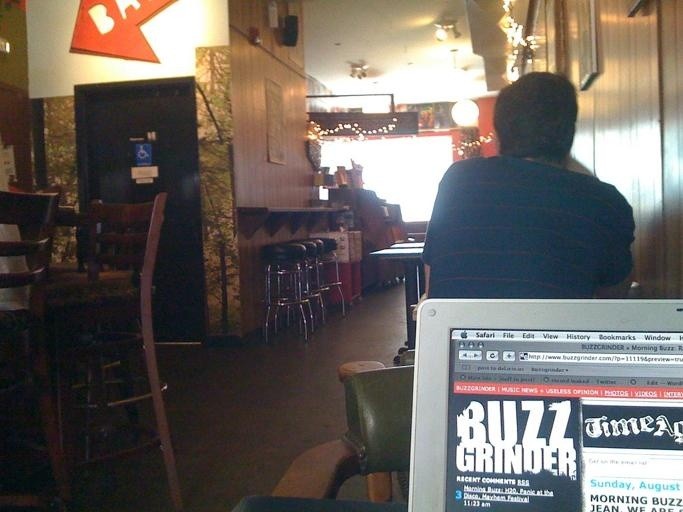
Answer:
[282,16,297,46]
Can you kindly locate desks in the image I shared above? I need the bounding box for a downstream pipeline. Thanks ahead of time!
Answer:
[366,231,436,366]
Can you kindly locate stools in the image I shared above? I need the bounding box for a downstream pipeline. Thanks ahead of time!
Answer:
[257,236,347,347]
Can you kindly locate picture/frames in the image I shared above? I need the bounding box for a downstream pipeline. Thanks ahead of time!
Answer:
[543,0,569,76]
[574,0,601,91]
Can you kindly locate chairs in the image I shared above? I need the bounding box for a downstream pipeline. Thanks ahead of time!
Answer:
[0,188,64,510]
[62,193,185,510]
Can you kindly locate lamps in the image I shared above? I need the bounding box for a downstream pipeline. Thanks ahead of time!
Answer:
[432,16,462,44]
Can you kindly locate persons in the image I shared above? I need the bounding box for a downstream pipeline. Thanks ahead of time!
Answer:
[422,72,636,299]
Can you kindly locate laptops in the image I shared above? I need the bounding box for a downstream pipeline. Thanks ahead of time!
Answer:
[408,298,683,511]
